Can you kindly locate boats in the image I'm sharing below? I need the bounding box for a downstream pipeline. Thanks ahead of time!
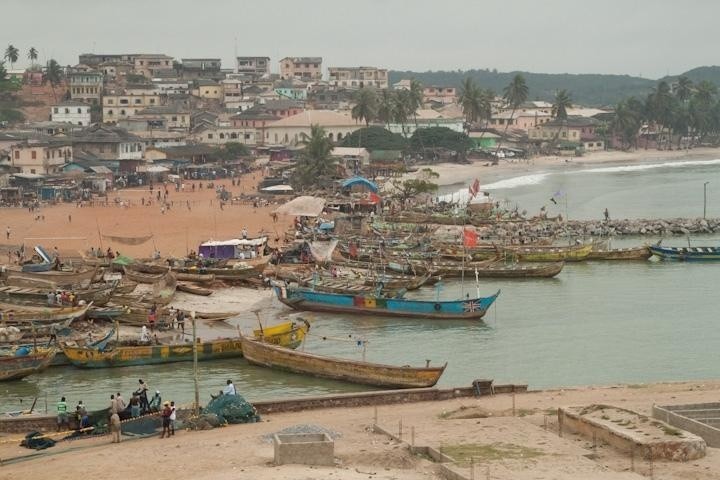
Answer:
[197,200,667,279]
[1,300,91,382]
[0,246,215,366]
[237,309,449,389]
[643,242,720,261]
[57,316,311,369]
[263,276,503,320]
[109,251,274,280]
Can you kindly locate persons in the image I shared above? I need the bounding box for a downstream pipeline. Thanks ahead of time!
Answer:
[210,380,236,398]
[48,286,80,306]
[48,319,60,346]
[142,301,184,344]
[2,161,610,276]
[57,379,176,443]
